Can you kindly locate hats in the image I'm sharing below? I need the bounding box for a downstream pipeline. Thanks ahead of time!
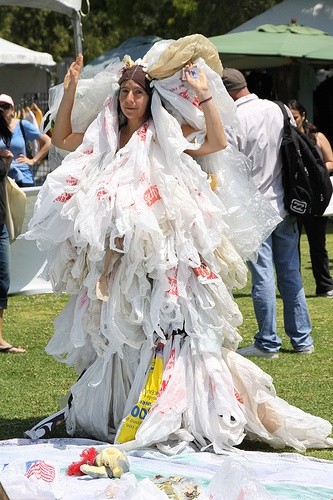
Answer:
[117,65,151,94]
[222,69,247,91]
[0,94,13,108]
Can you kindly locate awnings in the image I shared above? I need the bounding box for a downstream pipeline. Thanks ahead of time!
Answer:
[201,24,332,59]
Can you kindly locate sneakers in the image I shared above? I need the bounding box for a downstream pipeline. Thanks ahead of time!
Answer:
[236,344,279,358]
[294,344,315,355]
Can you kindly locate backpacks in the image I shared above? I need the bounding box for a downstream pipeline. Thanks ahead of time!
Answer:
[273,100,333,218]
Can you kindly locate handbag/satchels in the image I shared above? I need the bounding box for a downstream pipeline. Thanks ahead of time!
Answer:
[19,119,33,160]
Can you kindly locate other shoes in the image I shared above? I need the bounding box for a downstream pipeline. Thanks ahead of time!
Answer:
[316,289,333,298]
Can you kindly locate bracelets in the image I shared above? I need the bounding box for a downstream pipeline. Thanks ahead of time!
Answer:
[199,96,213,108]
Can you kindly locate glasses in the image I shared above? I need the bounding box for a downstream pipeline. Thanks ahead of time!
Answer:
[0,103,10,109]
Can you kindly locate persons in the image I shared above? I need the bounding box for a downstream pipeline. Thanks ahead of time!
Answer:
[0,93,52,355]
[218,67,333,357]
[52,54,229,443]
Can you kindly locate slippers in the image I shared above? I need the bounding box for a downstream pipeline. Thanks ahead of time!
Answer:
[0,343,26,353]
